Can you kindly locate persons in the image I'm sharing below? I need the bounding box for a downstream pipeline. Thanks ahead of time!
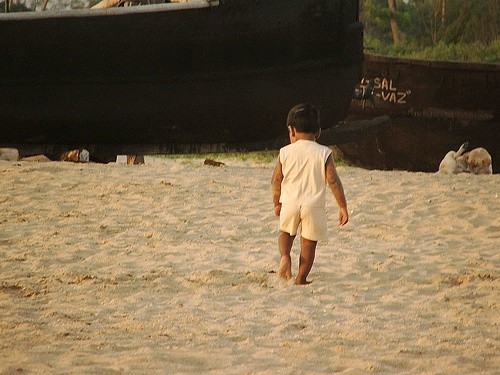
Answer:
[272,102,350,286]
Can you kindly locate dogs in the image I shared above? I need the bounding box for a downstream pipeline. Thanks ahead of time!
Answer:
[437,142,493,175]
